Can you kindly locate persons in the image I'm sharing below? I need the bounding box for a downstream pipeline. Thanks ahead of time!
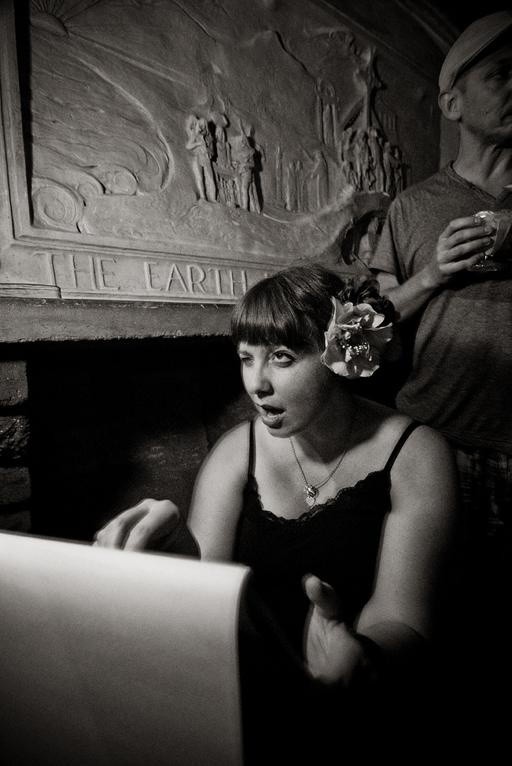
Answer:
[369,11,511,527]
[91,264,458,693]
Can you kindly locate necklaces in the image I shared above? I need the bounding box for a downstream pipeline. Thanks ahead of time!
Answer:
[288,434,346,507]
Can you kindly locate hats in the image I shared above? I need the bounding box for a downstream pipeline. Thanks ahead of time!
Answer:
[438,13,511,96]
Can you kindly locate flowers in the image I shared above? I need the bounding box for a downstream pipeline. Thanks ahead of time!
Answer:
[314,294,395,383]
